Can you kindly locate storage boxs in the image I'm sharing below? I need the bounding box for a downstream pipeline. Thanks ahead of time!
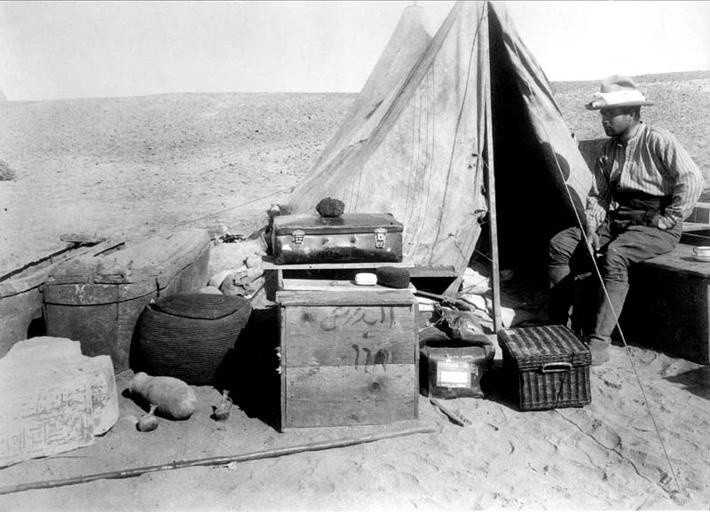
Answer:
[274,291,422,435]
[496,323,592,411]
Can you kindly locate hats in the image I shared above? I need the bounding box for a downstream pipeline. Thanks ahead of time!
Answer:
[584,75,654,111]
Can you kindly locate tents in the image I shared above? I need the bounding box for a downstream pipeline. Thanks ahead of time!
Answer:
[274,0,594,299]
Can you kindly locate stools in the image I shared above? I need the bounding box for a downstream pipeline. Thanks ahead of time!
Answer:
[628,242,709,368]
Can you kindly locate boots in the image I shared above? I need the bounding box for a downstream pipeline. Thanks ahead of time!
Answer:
[522,264,578,330]
[586,277,631,367]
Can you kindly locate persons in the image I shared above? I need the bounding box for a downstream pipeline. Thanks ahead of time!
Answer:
[519,75,705,367]
